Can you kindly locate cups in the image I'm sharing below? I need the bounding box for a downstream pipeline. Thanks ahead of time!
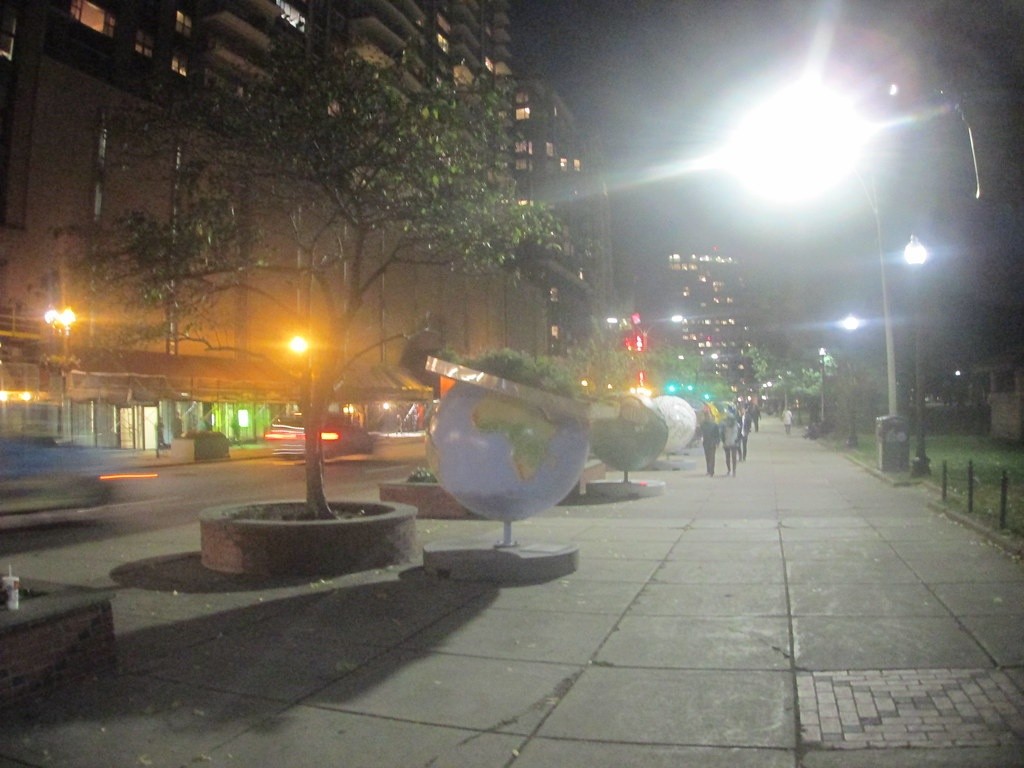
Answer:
[2,576,20,610]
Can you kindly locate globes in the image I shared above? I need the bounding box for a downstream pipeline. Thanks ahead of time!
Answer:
[653,396,697,455]
[590,392,669,483]
[420,356,590,549]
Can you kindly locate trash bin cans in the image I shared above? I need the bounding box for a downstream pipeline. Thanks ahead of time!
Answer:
[875,416,910,472]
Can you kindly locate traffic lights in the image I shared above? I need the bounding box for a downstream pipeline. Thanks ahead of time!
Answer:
[638,371,645,387]
[665,380,680,396]
[633,330,646,351]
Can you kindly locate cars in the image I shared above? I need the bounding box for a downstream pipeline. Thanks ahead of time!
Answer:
[268,409,372,462]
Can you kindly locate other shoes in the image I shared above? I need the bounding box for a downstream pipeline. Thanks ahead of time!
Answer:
[727,469,731,475]
[707,471,715,478]
[732,470,736,476]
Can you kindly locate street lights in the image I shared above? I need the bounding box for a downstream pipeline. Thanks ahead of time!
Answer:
[843,315,861,448]
[739,80,898,417]
[818,348,828,423]
[607,315,684,348]
[902,234,931,478]
[44,307,77,438]
